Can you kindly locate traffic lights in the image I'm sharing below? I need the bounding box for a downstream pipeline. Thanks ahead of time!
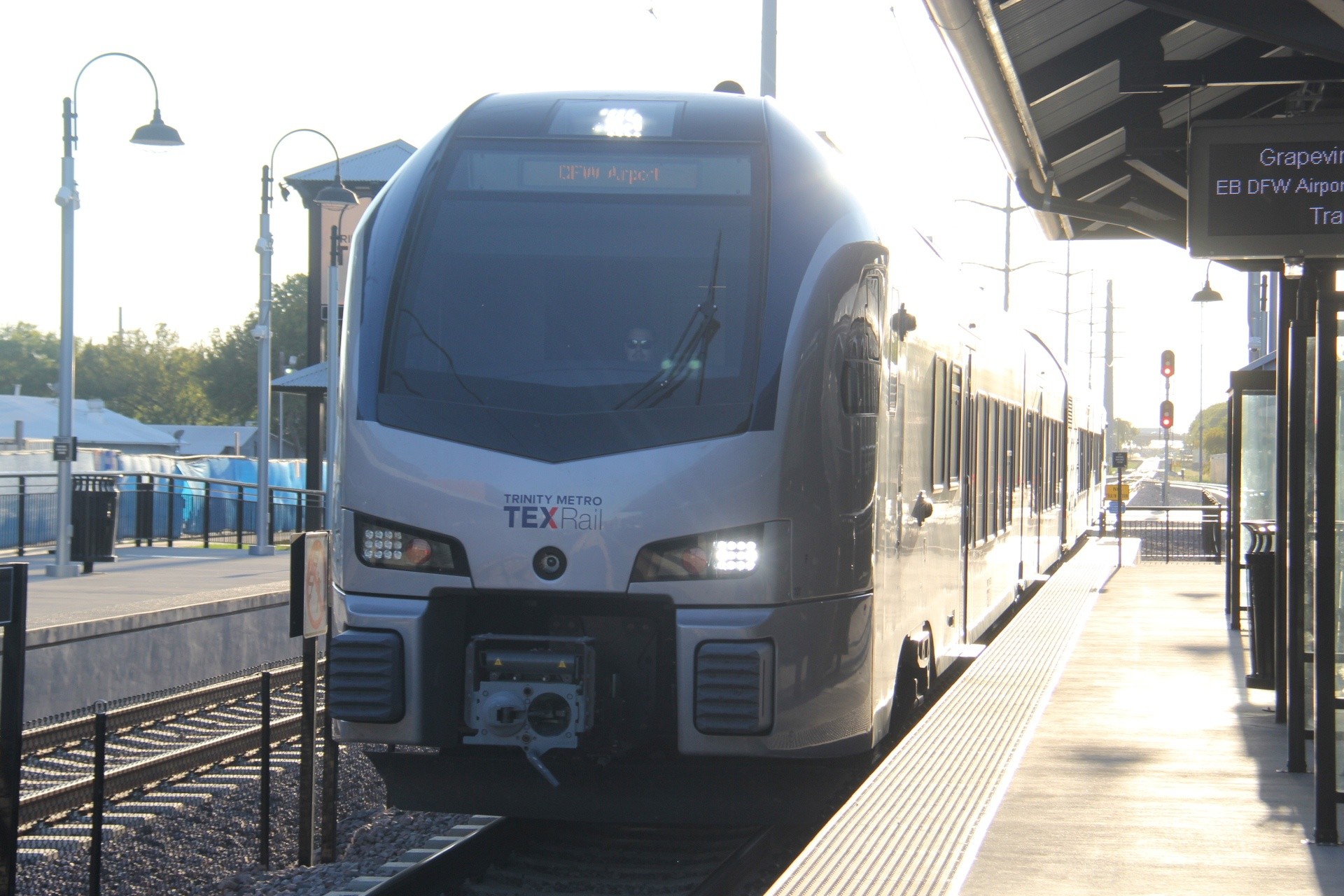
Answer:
[1160,349,1175,377]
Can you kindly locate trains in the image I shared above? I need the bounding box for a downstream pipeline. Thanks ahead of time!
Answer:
[326,81,1111,820]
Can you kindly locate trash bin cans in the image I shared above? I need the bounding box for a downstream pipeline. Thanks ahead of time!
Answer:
[70,472,124,574]
[1238,517,1277,692]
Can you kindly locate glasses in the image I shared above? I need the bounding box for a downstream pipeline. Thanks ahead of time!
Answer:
[626,339,650,349]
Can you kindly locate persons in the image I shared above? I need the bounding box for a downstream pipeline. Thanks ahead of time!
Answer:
[624,325,660,363]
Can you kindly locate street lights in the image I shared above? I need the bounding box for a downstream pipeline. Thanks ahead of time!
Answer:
[1190,260,1268,365]
[1159,400,1174,429]
[47,52,187,579]
[247,126,362,557]
